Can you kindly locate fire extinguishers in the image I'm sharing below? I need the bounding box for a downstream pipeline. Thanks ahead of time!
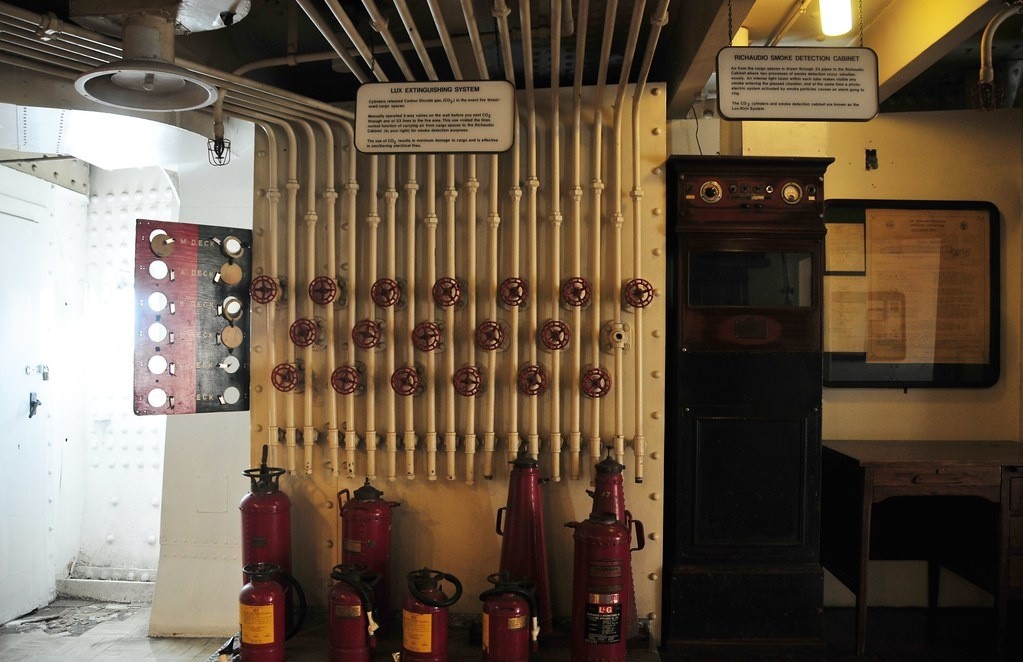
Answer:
[240,445,294,633]
[329,564,383,662]
[479,572,540,662]
[563,512,631,662]
[337,477,401,640]
[585,446,645,639]
[403,567,463,662]
[496,441,552,635]
[239,562,286,662]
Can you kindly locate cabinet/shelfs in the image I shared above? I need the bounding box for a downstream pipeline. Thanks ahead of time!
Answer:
[674,354,823,655]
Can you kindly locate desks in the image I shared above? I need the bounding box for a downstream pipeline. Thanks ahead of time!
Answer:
[822,439,1023,654]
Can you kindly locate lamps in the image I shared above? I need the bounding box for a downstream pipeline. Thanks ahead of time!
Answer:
[74,22,219,112]
[819,0,853,36]
